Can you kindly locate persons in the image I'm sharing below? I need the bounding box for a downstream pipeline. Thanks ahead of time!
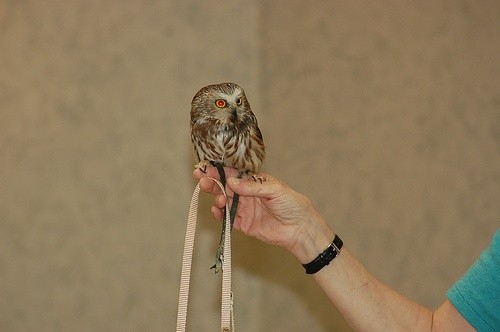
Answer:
[192,164,500,332]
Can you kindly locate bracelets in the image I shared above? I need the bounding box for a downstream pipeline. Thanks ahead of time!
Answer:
[301,234,343,274]
